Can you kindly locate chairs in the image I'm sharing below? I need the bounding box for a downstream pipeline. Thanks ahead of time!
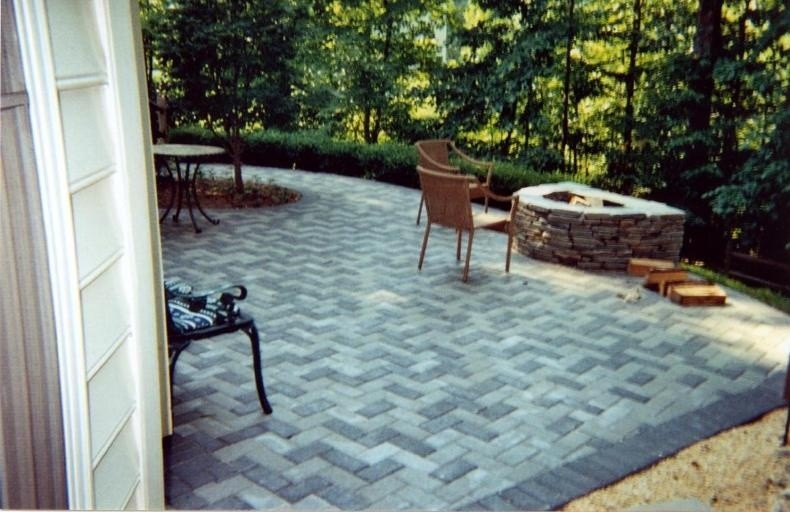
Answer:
[415,164,519,282]
[414,138,494,215]
[164,279,273,417]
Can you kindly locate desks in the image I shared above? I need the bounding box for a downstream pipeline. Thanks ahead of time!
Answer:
[150,142,224,234]
